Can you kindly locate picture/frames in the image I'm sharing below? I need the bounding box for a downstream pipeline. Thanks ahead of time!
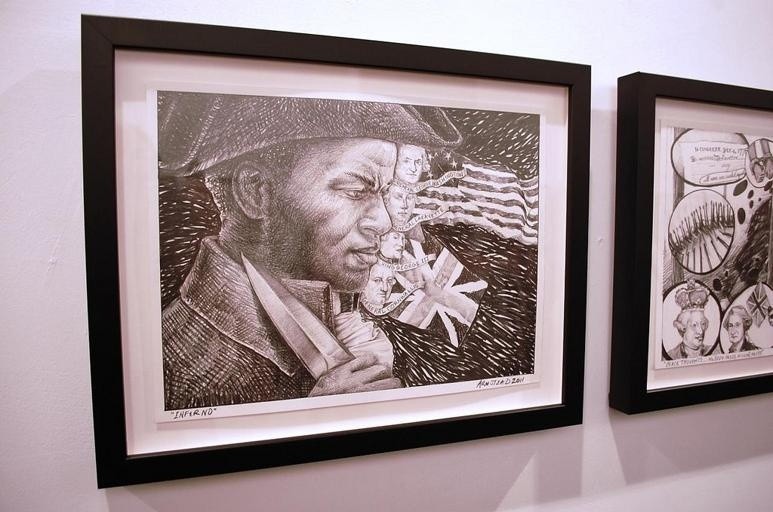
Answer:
[81,15,591,488]
[609,70,771,416]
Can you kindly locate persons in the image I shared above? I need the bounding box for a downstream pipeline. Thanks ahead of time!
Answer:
[394,143,430,187]
[382,177,417,228]
[667,278,712,360]
[379,230,407,264]
[747,139,772,186]
[722,304,763,354]
[362,258,395,310]
[155,89,403,413]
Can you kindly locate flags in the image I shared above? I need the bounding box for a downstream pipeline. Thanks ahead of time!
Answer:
[746,280,770,328]
[383,229,488,350]
[414,146,538,247]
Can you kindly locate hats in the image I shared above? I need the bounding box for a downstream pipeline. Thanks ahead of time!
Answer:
[158,90,465,178]
